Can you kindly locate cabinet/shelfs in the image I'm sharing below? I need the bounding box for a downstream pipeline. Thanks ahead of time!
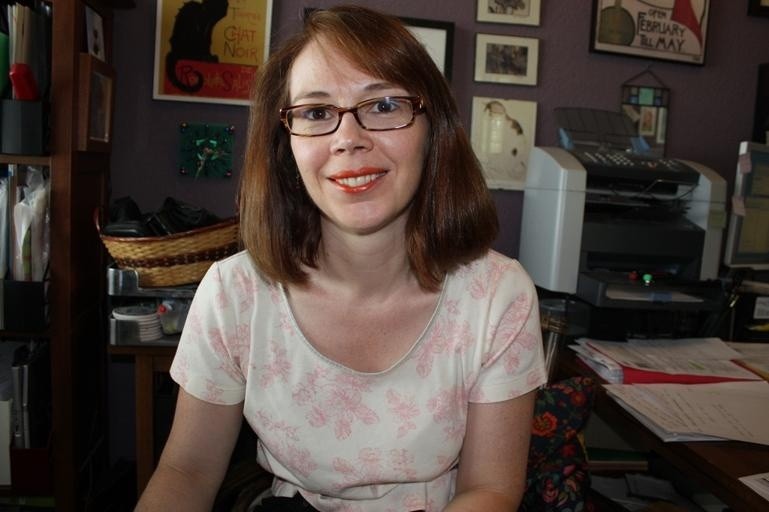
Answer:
[0,1,107,512]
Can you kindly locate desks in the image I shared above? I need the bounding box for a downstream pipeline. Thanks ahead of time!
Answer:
[107,330,269,512]
[563,348,769,512]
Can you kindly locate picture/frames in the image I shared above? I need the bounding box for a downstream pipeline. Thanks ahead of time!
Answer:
[589,0,712,67]
[475,0,543,27]
[83,4,108,63]
[472,32,541,87]
[747,0,769,19]
[394,16,455,87]
[151,0,274,108]
[77,52,114,153]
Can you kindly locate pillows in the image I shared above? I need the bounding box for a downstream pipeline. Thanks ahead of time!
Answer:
[517,376,602,512]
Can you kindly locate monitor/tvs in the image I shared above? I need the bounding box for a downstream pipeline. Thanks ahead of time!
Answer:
[722,140,769,296]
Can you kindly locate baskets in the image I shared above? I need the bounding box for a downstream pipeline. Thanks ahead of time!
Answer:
[92,210,243,287]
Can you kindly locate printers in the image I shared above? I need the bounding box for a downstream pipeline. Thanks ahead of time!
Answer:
[518,107,728,312]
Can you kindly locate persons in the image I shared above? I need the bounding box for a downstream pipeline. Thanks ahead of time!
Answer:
[134,6,550,511]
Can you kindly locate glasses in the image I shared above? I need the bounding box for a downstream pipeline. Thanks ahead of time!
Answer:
[279,95,425,137]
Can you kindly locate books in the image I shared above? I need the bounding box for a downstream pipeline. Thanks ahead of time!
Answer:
[566,331,769,445]
[22,349,50,450]
[10,346,27,449]
